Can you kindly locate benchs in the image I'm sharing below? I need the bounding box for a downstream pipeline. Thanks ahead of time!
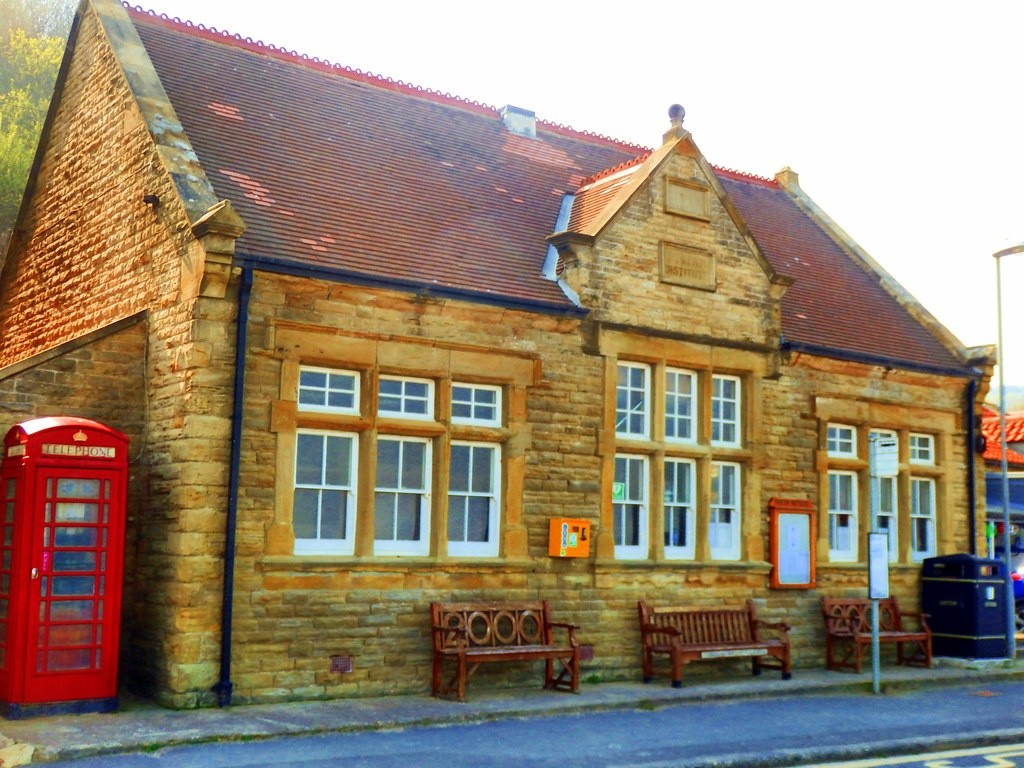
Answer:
[637,598,794,688]
[820,593,933,675]
[428,598,582,704]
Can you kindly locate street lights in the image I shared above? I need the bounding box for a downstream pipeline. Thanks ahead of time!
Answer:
[992,244,1023,662]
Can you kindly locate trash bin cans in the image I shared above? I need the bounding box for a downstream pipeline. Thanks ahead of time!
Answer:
[919,552,1006,658]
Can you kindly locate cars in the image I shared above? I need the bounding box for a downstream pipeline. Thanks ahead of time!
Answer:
[988,471,1023,599]
